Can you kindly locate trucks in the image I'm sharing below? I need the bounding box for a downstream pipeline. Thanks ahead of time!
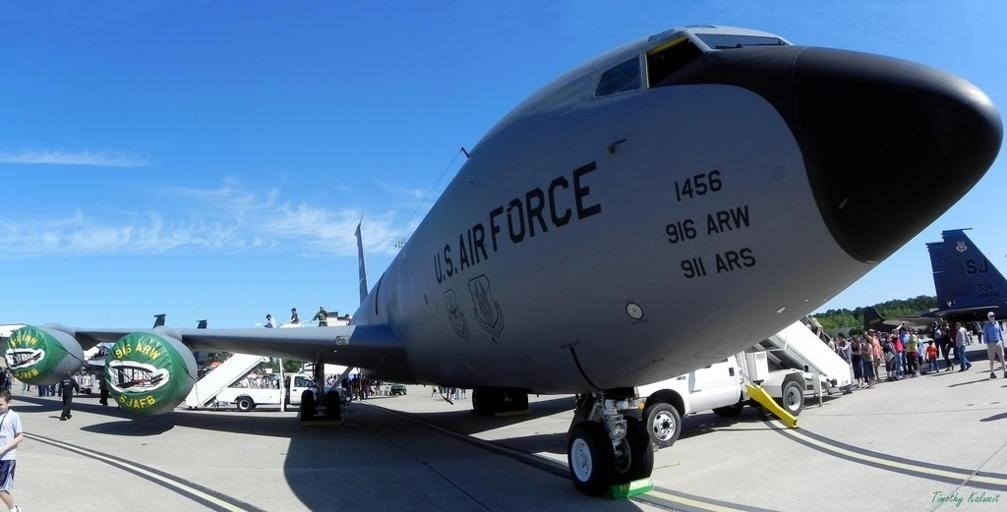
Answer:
[604,316,853,449]
[185,353,347,412]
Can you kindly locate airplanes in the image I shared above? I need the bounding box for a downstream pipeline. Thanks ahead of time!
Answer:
[0,23,1004,496]
[863,228,1007,335]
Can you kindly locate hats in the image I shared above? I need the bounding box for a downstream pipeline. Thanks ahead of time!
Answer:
[868,329,876,332]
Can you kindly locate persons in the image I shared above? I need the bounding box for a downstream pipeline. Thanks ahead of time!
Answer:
[289,307,300,324]
[284,372,384,400]
[56,371,80,420]
[311,305,329,327]
[345,313,353,324]
[0,388,24,512]
[0,367,109,406]
[800,311,1006,390]
[430,385,466,400]
[265,314,277,328]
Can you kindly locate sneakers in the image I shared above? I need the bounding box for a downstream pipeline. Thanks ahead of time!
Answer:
[990,372,1007,378]
[855,365,971,388]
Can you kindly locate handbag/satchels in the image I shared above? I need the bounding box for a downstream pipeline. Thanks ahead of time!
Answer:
[884,351,894,362]
